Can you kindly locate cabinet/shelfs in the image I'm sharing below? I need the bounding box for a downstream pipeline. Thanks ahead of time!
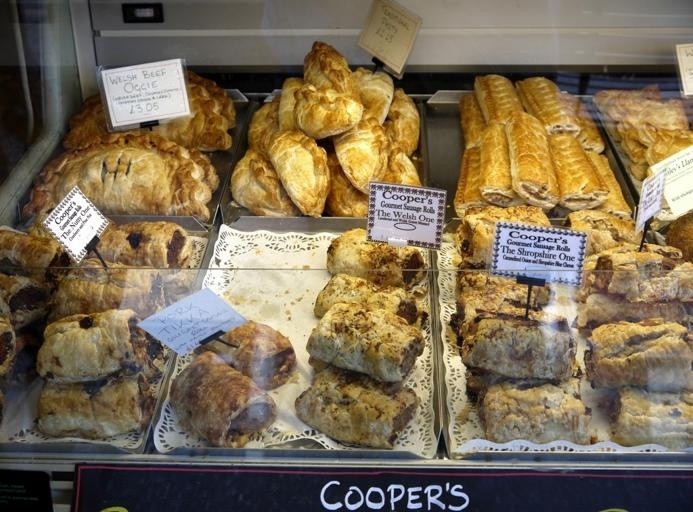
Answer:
[0,0,693,511]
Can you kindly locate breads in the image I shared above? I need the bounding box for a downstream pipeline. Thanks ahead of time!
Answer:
[230,42,420,218]
[170,227,447,448]
[454,72,633,217]
[448,204,692,444]
[0,211,195,436]
[596,82,693,176]
[25,70,237,219]
